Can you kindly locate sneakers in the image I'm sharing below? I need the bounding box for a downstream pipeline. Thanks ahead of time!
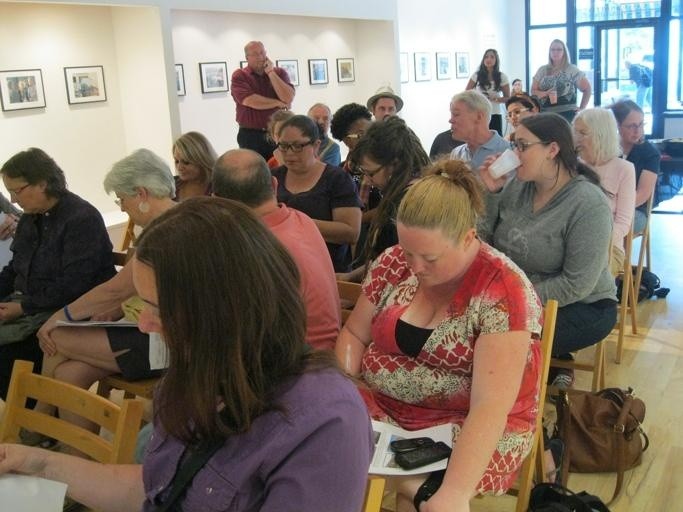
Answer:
[547,374,573,403]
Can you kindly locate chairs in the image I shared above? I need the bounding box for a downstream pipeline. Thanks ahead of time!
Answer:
[97,372,160,398]
[337,281,362,325]
[362,477,385,512]
[547,192,652,394]
[114,217,144,265]
[1,359,146,467]
[506,299,558,512]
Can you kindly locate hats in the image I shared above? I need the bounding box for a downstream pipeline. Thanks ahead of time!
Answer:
[367,87,403,113]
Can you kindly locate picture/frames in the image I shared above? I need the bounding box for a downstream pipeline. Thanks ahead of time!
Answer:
[199,61,229,94]
[64,64,109,105]
[1,69,48,113]
[276,59,300,87]
[402,49,472,83]
[240,60,248,69]
[308,59,329,85]
[174,64,187,97]
[336,58,355,83]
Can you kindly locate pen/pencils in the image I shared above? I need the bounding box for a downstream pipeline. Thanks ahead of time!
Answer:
[345,342,351,372]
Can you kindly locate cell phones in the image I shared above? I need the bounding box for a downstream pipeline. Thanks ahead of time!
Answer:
[394,441,452,470]
[392,437,432,451]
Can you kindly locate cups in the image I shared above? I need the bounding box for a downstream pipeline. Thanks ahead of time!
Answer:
[487,148,523,180]
[548,90,557,105]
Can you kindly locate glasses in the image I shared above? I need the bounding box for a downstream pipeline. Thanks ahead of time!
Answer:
[8,184,30,196]
[622,121,647,130]
[346,133,364,139]
[278,140,313,152]
[510,139,551,152]
[505,108,530,119]
[358,165,384,177]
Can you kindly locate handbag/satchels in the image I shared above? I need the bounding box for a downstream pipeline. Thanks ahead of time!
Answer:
[615,268,660,304]
[550,387,646,473]
[526,482,610,512]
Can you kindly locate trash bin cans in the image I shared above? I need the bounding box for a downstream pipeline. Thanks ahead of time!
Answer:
[663,139,683,174]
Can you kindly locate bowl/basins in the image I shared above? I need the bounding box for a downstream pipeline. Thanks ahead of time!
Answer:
[663,138,683,158]
